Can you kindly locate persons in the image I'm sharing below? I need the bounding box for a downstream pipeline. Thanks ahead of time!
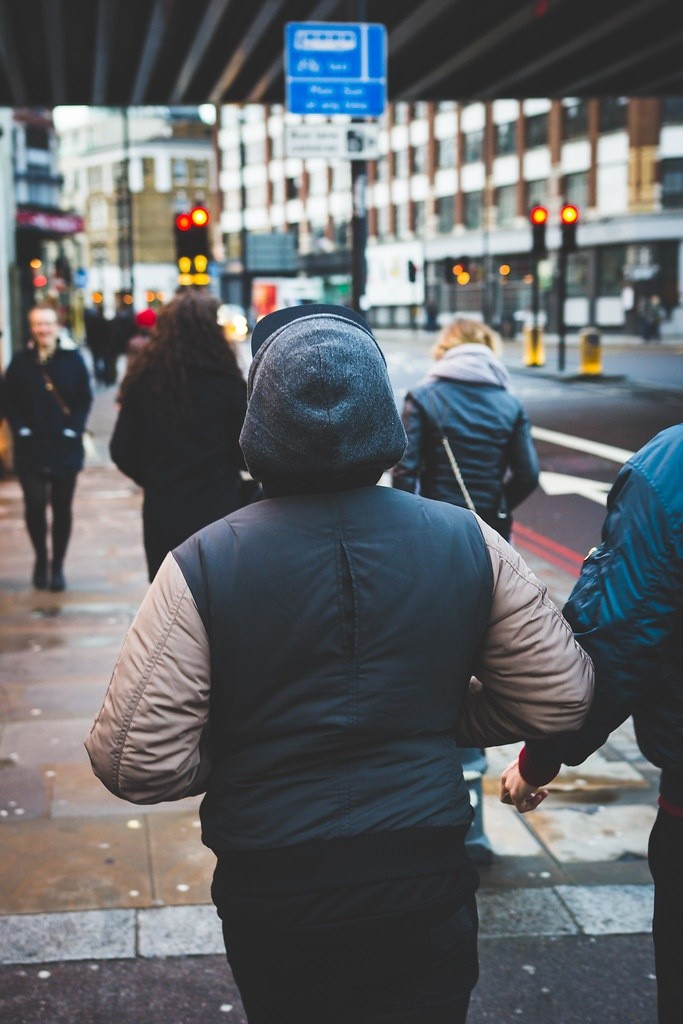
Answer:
[642,295,666,345]
[0,289,247,592]
[84,305,594,1024]
[500,424,683,1024]
[392,316,540,864]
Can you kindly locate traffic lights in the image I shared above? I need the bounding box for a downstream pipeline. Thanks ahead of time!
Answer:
[529,205,552,259]
[174,213,197,277]
[561,206,580,255]
[191,205,214,267]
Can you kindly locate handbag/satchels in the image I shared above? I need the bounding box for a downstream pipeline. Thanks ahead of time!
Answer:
[79,431,98,460]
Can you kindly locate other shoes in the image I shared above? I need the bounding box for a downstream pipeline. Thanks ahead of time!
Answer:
[50,562,66,591]
[34,556,47,588]
